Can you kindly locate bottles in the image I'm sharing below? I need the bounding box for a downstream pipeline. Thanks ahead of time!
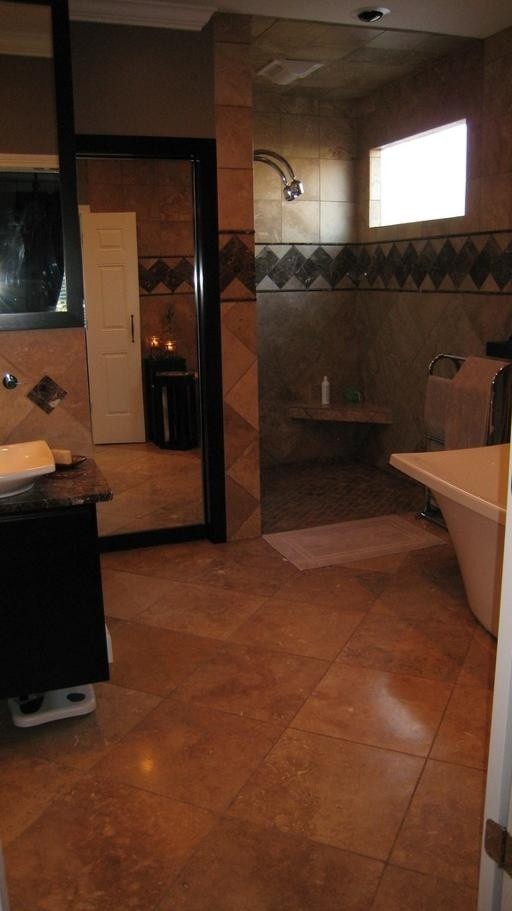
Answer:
[321,375,331,405]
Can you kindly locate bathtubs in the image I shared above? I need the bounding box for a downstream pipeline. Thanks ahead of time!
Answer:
[388,442,509,642]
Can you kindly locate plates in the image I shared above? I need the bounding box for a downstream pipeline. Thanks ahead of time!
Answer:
[53,455,87,468]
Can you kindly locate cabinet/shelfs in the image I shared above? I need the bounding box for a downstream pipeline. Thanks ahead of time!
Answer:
[0,504,113,728]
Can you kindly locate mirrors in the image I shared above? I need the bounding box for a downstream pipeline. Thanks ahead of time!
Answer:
[76,137,226,555]
[1,153,83,333]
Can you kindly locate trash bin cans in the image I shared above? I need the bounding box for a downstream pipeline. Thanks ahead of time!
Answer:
[143,370,197,451]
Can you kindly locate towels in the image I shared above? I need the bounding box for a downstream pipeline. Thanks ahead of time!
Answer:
[445,355,509,454]
[422,375,450,444]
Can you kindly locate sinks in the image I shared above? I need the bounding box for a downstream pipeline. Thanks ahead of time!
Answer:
[0,438,58,498]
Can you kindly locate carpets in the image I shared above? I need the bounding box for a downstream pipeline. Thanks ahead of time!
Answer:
[263,514,447,572]
